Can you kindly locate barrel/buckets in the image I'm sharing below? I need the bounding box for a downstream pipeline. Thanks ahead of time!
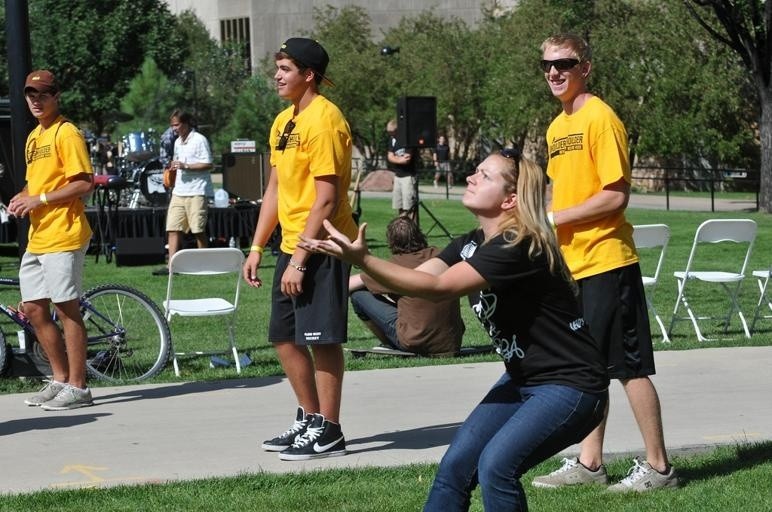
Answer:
[215,188,229,209]
[17,330,53,382]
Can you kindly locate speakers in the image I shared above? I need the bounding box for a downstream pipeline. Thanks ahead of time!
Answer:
[221,153,266,204]
[396,94,439,149]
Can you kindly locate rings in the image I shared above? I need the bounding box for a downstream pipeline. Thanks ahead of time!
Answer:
[175,165,178,167]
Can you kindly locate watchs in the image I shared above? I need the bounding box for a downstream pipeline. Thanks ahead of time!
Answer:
[184,163,189,170]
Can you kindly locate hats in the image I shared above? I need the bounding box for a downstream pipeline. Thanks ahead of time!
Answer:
[23,69,61,98]
[279,36,338,90]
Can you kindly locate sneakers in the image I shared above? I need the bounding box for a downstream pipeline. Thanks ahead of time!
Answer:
[530,455,610,491]
[23,379,67,407]
[605,456,681,496]
[261,405,315,452]
[40,383,95,411]
[151,266,180,277]
[278,413,348,460]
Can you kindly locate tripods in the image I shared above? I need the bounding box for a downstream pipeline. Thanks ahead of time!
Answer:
[393,151,453,239]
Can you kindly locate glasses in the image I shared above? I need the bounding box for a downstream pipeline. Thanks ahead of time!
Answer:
[540,56,587,75]
[278,117,296,150]
[500,147,522,173]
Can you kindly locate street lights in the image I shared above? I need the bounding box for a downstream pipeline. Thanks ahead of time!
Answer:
[182,69,199,129]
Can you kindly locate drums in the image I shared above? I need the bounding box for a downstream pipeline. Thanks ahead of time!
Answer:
[131,156,173,206]
[122,128,160,162]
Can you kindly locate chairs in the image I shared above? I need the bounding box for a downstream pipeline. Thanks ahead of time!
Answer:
[750,269,772,337]
[631,223,678,344]
[155,244,252,379]
[662,214,760,344]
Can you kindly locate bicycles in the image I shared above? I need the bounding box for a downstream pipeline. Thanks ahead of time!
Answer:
[0,203,174,387]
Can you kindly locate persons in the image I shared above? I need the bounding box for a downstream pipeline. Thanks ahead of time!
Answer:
[7,70,95,412]
[151,109,213,274]
[159,116,197,199]
[386,120,417,218]
[433,136,453,189]
[242,38,359,461]
[349,216,466,356]
[530,33,680,495]
[295,152,611,511]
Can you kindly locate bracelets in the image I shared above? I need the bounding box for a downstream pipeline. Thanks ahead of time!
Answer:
[250,246,264,254]
[288,259,307,271]
[545,211,559,230]
[40,192,49,206]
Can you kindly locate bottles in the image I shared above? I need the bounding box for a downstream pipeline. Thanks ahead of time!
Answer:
[118,126,159,155]
[229,235,236,247]
